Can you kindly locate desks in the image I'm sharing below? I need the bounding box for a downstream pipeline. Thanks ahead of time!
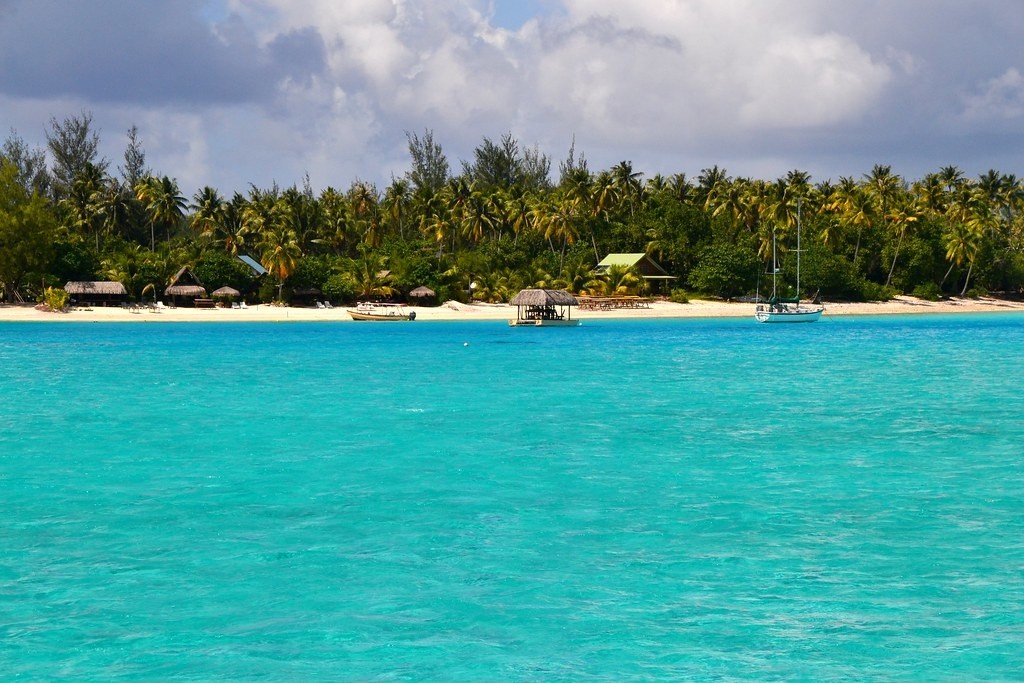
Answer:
[590,296,606,306]
[607,295,624,305]
[624,296,639,308]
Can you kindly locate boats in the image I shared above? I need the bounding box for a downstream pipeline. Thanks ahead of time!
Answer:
[509,289,579,327]
[346,303,416,321]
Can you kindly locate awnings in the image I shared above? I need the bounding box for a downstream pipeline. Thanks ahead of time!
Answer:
[642,276,679,288]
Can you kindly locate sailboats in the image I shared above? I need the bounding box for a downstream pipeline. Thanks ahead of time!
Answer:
[754,198,824,323]
[787,309,796,312]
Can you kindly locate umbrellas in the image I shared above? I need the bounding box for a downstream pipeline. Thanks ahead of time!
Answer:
[212,286,240,303]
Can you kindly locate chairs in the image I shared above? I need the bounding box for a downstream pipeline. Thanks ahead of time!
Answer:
[316,302,325,309]
[121,302,128,309]
[325,301,333,309]
[157,301,166,309]
[231,302,240,309]
[557,310,565,320]
[579,299,649,312]
[168,302,177,309]
[129,302,139,313]
[240,302,248,309]
[148,302,161,312]
[357,302,364,309]
[138,302,147,309]
[136,304,143,309]
